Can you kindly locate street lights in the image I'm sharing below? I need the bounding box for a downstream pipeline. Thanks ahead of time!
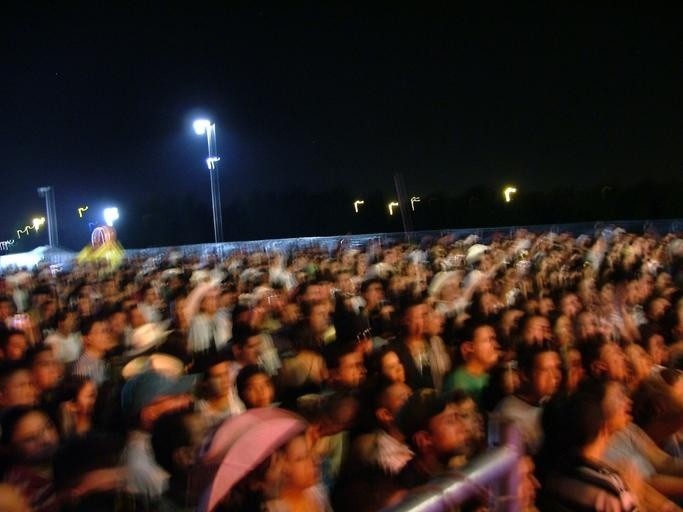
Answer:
[192,118,226,243]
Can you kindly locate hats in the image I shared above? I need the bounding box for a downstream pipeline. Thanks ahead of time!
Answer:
[467,244,489,263]
[128,322,167,354]
[427,270,464,296]
[120,370,201,421]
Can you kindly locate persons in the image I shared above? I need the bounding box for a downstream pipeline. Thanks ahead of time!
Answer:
[0,222,683,512]
